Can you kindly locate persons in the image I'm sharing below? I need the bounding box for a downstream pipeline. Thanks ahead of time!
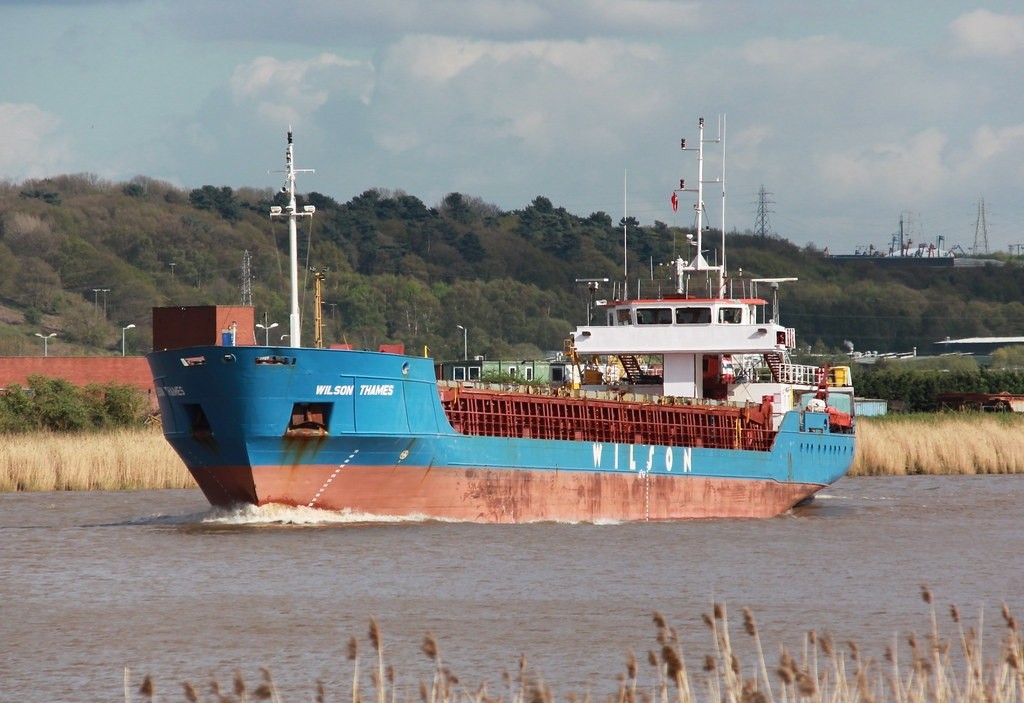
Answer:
[637,311,644,323]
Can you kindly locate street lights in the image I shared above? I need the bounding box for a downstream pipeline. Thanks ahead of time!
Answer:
[34,332,57,357]
[457,325,468,360]
[122,324,136,357]
[256,323,278,346]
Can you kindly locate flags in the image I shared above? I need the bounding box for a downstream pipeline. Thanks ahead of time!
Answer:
[671,193,678,212]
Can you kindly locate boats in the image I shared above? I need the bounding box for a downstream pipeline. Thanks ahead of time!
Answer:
[142,112,858,524]
